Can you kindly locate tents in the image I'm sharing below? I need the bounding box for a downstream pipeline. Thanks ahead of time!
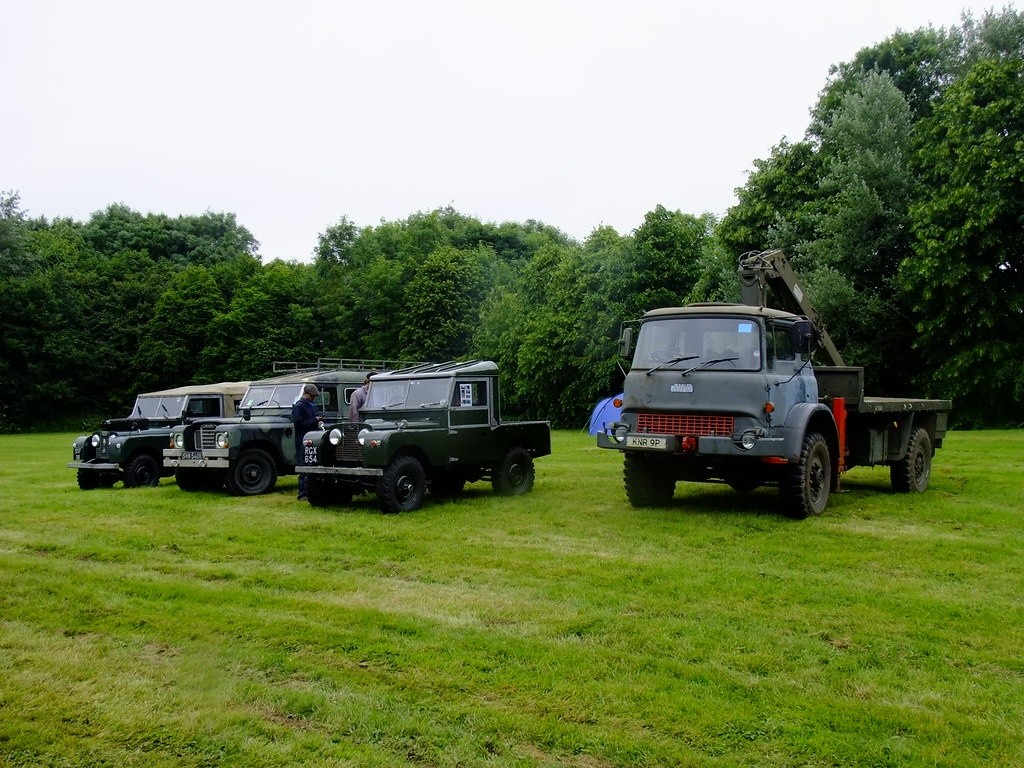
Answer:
[588,392,624,435]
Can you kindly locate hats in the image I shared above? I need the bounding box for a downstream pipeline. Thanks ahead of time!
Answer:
[303,384,320,397]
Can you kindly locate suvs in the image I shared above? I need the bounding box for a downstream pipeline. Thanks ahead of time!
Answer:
[163,357,435,496]
[66,381,254,491]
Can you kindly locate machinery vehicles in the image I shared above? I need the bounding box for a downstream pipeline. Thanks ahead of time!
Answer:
[596,248,952,522]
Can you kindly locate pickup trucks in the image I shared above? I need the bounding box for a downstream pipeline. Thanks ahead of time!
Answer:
[294,359,551,514]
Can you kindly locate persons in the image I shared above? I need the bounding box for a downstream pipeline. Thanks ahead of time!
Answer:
[350,372,379,496]
[291,385,323,500]
